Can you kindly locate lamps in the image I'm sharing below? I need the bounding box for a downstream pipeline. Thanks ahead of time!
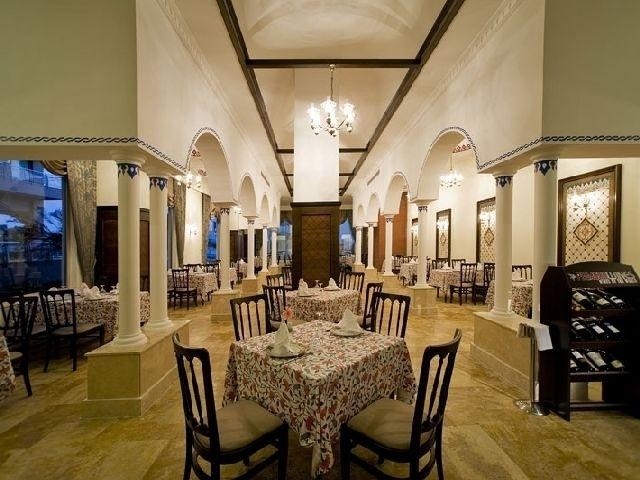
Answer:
[306,64,357,134]
[439,153,465,191]
[569,192,591,211]
[186,171,202,190]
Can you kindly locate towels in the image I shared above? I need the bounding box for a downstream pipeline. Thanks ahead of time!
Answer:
[298,282,309,295]
[272,321,292,354]
[338,307,361,333]
[328,277,337,288]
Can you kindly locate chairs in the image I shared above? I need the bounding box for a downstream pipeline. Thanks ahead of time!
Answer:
[452,258,466,270]
[139,276,151,293]
[356,281,384,331]
[172,269,197,310]
[188,264,202,272]
[229,294,271,341]
[167,287,174,309]
[0,295,45,366]
[1,296,39,396]
[169,332,291,479]
[183,265,196,272]
[281,266,292,290]
[261,284,287,333]
[266,274,286,313]
[344,271,364,293]
[204,264,219,286]
[476,263,496,304]
[39,289,104,370]
[511,265,532,282]
[484,265,496,289]
[367,292,411,338]
[339,328,462,480]
[435,258,450,268]
[451,263,476,305]
[339,264,352,290]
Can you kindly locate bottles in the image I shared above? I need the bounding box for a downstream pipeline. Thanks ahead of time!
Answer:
[585,291,610,308]
[597,289,624,308]
[572,290,596,311]
[578,315,605,338]
[571,348,591,371]
[569,359,579,373]
[604,350,624,371]
[600,315,621,338]
[584,347,607,371]
[570,317,590,338]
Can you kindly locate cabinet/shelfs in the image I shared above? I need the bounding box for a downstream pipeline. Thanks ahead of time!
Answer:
[540,261,640,423]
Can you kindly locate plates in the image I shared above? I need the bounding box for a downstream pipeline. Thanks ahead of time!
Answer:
[297,293,314,297]
[266,342,306,359]
[330,329,362,337]
[324,287,340,291]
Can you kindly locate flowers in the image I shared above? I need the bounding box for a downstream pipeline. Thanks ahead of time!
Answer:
[277,303,294,321]
